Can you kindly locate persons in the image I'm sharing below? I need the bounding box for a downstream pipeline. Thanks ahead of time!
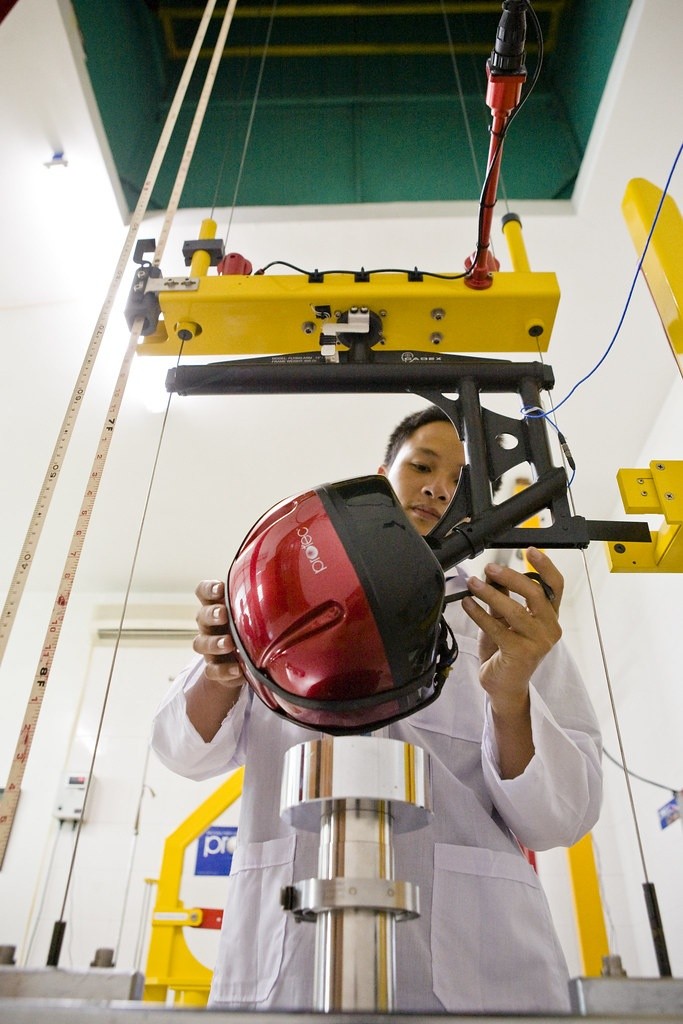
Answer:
[150,405,604,1014]
